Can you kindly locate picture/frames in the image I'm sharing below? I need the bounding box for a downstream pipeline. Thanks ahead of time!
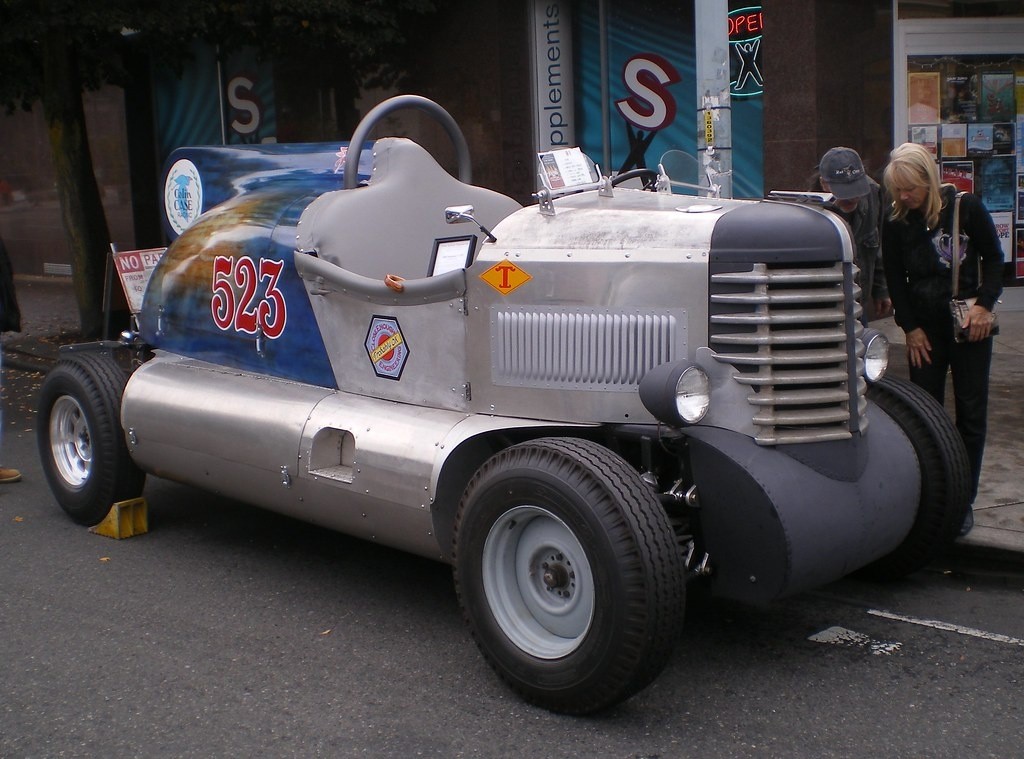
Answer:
[426,235,477,278]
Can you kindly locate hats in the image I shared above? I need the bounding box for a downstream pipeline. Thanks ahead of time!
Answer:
[819,145,870,199]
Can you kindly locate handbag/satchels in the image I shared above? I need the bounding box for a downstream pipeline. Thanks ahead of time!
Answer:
[948,296,1002,342]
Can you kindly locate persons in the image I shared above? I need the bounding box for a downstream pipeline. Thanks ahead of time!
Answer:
[881,142,1005,532]
[0,238,21,481]
[808,146,891,329]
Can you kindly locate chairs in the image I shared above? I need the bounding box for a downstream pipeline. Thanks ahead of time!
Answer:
[295,137,524,412]
